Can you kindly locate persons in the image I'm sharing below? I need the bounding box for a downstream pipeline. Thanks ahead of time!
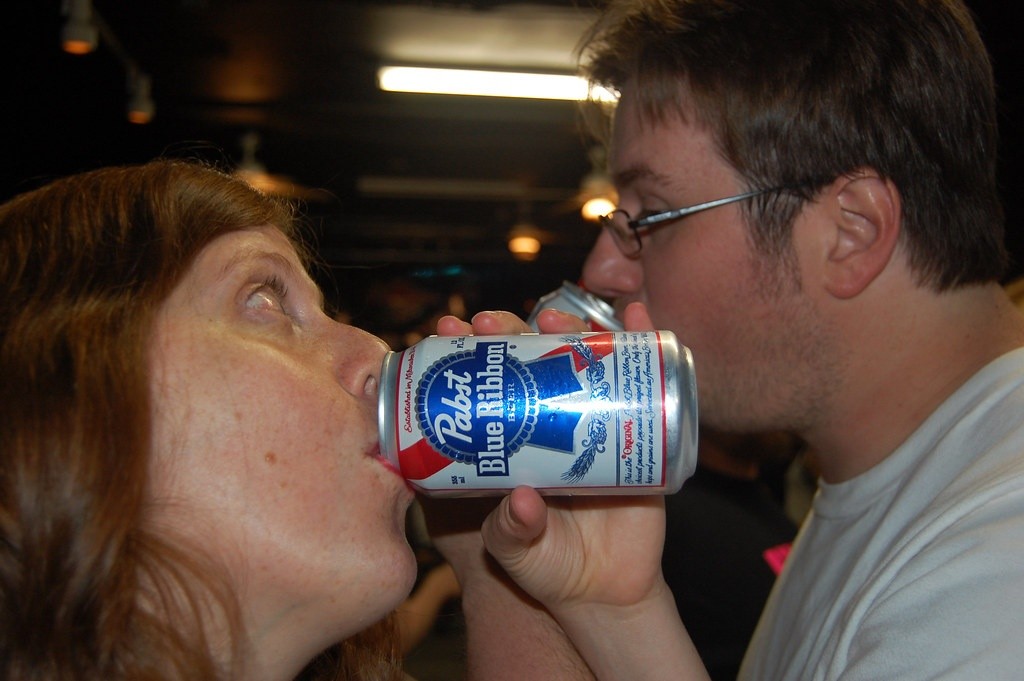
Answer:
[412,0,1024,681]
[0,160,714,681]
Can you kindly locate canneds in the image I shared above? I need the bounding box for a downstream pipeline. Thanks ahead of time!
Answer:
[527,280,628,332]
[377,333,699,499]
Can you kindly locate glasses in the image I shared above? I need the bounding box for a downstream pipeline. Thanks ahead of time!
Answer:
[596,185,787,258]
